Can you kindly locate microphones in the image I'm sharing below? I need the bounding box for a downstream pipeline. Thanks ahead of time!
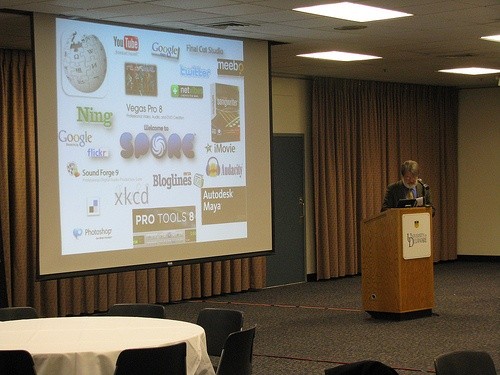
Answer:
[417,178,429,190]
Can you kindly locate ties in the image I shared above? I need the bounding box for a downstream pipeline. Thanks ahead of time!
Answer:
[406,190,413,199]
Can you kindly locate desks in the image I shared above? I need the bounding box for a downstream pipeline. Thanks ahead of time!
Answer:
[0,317,216,375]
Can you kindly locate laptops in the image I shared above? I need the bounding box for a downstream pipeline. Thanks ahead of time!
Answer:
[396,199,416,208]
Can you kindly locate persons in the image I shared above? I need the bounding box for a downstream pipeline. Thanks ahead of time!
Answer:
[381,160,436,217]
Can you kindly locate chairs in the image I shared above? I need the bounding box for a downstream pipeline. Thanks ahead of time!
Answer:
[196,308,243,373]
[115,342,187,375]
[216,325,256,375]
[434,349,497,375]
